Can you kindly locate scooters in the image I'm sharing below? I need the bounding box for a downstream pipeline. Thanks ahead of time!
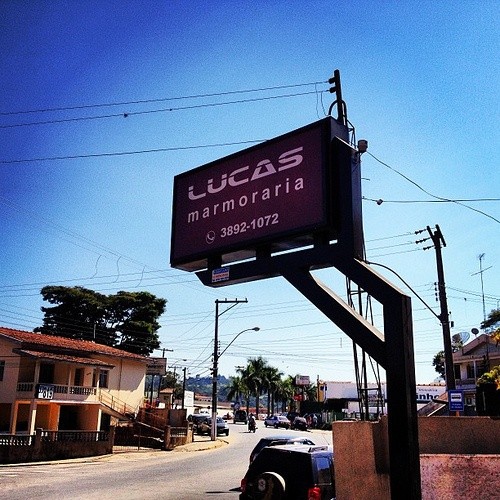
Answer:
[248,422,258,433]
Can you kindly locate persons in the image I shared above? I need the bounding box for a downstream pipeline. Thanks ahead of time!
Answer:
[248,413,258,433]
[306,413,312,429]
[312,413,318,429]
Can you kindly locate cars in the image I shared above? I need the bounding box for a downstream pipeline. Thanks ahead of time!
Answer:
[293,416,308,431]
[187,413,230,436]
[264,416,291,430]
[249,434,316,468]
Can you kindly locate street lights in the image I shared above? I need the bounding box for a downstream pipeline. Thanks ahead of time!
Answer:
[210,327,261,442]
[157,359,187,398]
[363,259,458,416]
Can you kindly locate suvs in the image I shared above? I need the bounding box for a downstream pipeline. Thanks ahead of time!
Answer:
[232,410,248,424]
[240,438,337,500]
